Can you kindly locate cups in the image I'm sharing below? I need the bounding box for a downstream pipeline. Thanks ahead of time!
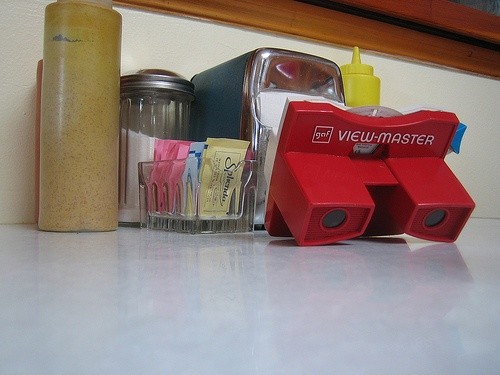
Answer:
[38,0,123,232]
[119,64,193,230]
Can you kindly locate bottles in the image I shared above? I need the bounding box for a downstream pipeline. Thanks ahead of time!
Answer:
[339,44,381,112]
